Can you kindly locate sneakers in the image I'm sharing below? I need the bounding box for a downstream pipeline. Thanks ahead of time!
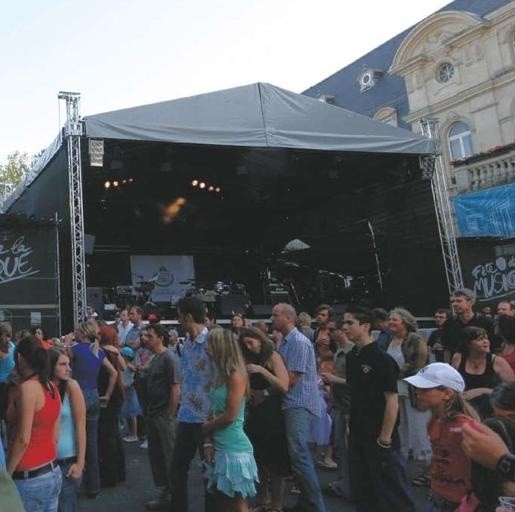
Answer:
[320,458,337,470]
[145,499,169,508]
[140,440,148,448]
[121,436,138,442]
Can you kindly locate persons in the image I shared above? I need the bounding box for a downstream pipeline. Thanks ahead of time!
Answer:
[32,325,51,352]
[404,363,481,512]
[496,314,515,367]
[46,348,86,512]
[373,309,392,351]
[386,306,433,512]
[146,323,180,508]
[237,327,290,512]
[126,306,146,346]
[483,306,491,314]
[314,304,337,373]
[299,312,313,340]
[118,308,133,344]
[205,312,216,325]
[232,313,245,330]
[94,328,126,487]
[483,378,515,512]
[450,326,515,390]
[119,347,142,442]
[322,314,354,496]
[0,322,16,469]
[441,288,493,365]
[84,304,99,322]
[272,303,327,512]
[203,328,259,512]
[67,321,117,500]
[20,329,31,338]
[7,337,63,511]
[174,296,216,511]
[137,328,153,447]
[457,420,515,484]
[169,330,183,351]
[497,300,515,317]
[342,308,416,512]
[427,309,447,367]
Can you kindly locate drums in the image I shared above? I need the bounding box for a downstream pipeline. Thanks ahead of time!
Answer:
[214,281,224,292]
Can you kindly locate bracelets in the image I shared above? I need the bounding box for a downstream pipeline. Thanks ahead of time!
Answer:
[204,443,215,448]
[377,438,392,448]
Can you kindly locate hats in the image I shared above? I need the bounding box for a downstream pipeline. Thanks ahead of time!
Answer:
[402,362,465,393]
[120,347,133,357]
[168,329,178,336]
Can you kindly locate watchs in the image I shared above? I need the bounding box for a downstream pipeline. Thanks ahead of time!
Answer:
[496,451,515,480]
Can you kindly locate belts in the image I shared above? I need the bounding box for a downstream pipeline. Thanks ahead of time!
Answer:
[13,457,58,479]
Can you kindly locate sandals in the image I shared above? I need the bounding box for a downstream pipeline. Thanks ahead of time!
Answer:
[412,475,425,486]
